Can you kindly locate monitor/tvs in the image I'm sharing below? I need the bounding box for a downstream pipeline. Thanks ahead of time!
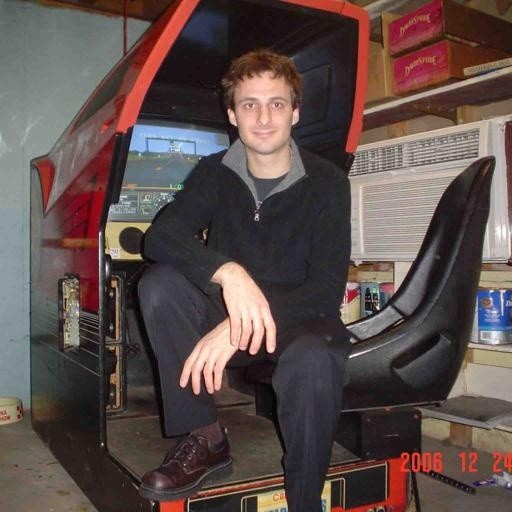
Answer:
[108,113,238,222]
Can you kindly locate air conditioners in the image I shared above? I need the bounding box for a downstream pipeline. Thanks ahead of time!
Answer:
[347,119,510,264]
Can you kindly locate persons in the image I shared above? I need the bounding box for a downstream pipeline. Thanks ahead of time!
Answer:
[134,49,350,511]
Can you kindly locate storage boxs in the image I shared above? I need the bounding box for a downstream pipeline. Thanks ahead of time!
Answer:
[388,0,512,59]
[391,40,512,97]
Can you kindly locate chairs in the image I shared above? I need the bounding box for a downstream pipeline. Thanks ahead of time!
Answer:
[227,155,496,512]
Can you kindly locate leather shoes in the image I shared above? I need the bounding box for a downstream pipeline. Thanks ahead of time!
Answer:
[138,427,233,499]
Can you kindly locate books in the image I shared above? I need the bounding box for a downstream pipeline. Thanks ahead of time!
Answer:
[416,392,512,431]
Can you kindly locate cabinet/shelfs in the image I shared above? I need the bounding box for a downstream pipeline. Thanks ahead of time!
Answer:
[359,0,512,125]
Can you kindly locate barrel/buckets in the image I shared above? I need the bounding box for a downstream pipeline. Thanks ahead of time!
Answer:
[469,287,512,345]
[339,282,361,325]
